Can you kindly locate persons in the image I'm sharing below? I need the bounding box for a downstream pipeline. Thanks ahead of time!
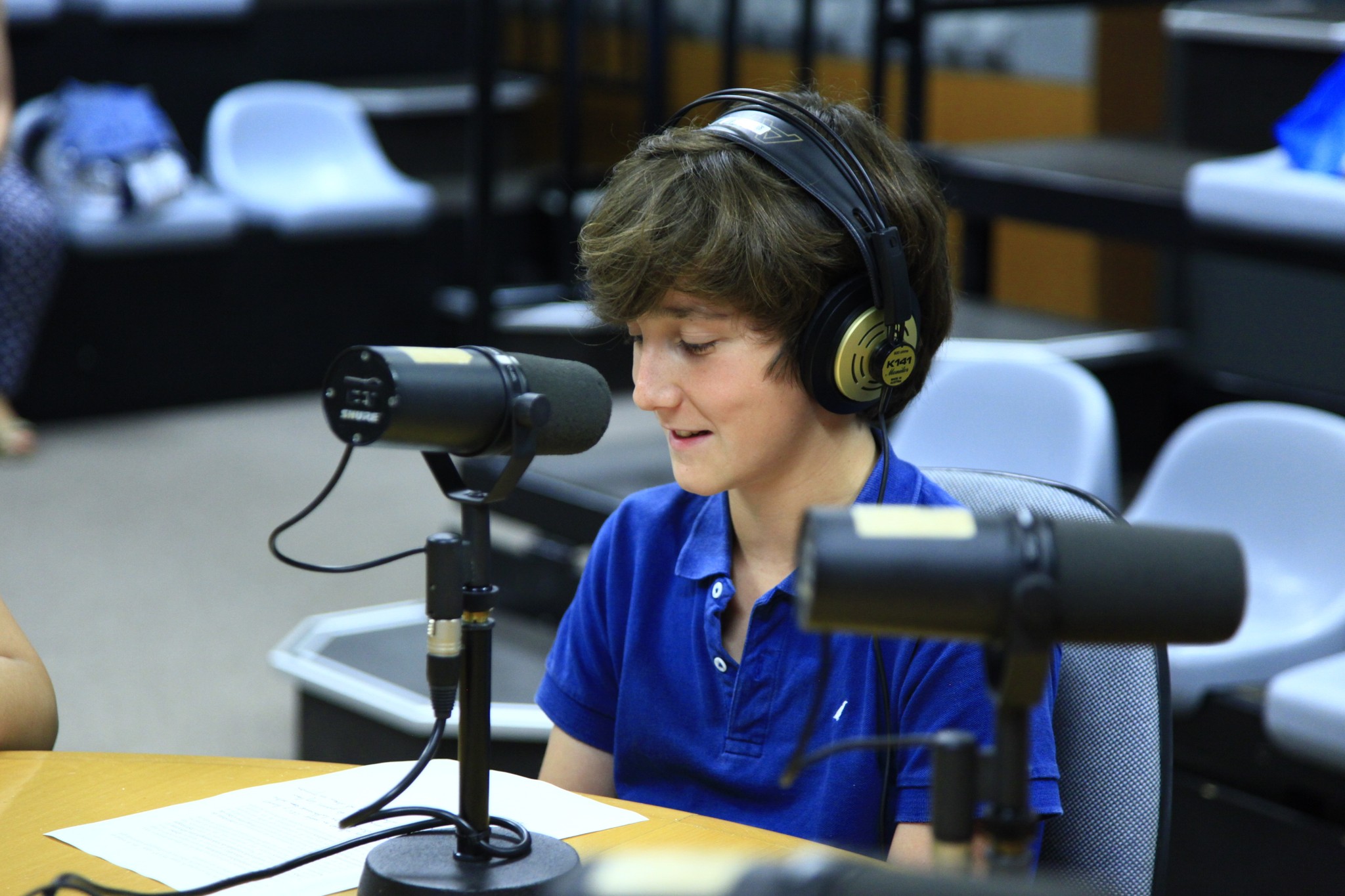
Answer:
[0,10,60,456]
[0,598,60,753]
[528,90,1067,885]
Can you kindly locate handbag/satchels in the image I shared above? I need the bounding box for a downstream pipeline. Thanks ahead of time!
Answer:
[50,85,189,231]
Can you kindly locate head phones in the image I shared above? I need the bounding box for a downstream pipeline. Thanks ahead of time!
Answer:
[658,88,928,415]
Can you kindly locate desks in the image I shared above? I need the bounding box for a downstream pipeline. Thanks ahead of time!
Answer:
[929,144,1345,394]
[0,753,877,896]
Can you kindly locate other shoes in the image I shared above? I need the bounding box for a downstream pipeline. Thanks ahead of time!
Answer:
[2,416,43,460]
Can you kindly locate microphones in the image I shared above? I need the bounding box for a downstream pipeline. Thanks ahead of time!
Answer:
[323,344,613,458]
[791,507,1249,648]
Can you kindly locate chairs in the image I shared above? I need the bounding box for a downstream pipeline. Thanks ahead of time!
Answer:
[473,337,1345,896]
[13,83,438,421]
